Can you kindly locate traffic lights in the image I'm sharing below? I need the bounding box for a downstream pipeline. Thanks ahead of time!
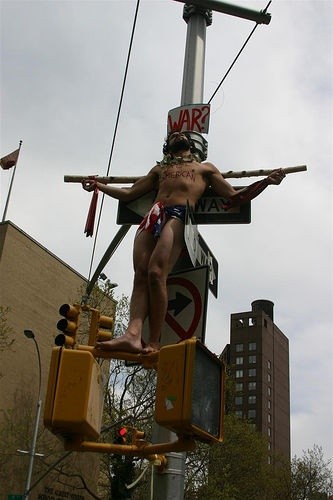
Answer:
[154,336,226,445]
[54,303,78,348]
[115,425,127,445]
[92,310,114,342]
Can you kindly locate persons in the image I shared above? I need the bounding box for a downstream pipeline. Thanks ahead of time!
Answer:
[82,129,286,354]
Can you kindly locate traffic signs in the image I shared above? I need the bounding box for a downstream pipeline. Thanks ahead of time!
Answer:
[116,184,251,226]
[183,199,219,300]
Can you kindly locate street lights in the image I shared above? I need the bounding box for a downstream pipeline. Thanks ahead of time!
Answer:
[19,329,43,498]
